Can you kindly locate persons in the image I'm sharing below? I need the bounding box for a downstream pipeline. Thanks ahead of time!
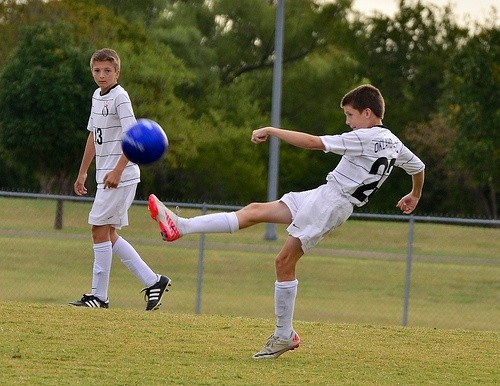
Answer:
[147,83,426,362]
[72,48,171,312]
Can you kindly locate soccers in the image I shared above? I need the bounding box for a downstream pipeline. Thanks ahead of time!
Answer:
[121,118,169,165]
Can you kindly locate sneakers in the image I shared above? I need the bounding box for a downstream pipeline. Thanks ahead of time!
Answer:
[139,274,173,311]
[69,294,110,310]
[148,194,183,241]
[252,329,301,359]
